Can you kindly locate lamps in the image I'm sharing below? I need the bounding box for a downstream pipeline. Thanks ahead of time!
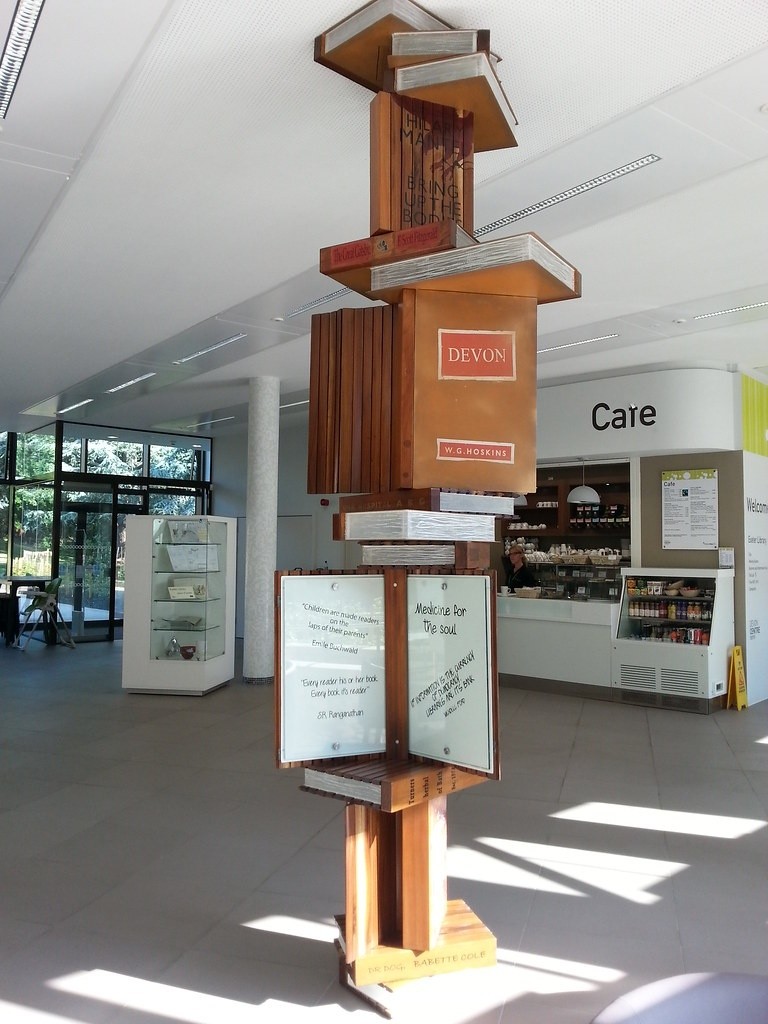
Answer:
[273,318,283,321]
[178,332,248,364]
[567,466,600,504]
[285,288,353,318]
[58,397,94,414]
[536,334,619,354]
[104,371,156,393]
[675,319,686,323]
[172,362,180,365]
[471,154,661,237]
[693,301,768,320]
[760,105,768,114]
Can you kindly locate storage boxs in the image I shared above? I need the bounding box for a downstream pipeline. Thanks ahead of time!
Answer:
[562,554,588,565]
[515,587,539,598]
[590,554,622,564]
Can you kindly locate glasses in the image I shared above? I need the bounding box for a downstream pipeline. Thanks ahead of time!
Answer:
[507,551,522,557]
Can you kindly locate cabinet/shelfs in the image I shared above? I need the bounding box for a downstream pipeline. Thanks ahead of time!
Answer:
[611,566,737,714]
[122,515,238,696]
[500,478,630,591]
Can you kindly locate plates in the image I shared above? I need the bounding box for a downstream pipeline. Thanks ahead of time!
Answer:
[497,593,512,597]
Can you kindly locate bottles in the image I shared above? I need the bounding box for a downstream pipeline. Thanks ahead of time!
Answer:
[628,599,713,620]
[638,624,710,647]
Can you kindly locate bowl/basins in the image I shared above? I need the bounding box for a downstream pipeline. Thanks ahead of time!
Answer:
[664,579,684,596]
[678,587,698,596]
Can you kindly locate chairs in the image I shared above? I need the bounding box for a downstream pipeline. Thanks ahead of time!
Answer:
[13,578,76,651]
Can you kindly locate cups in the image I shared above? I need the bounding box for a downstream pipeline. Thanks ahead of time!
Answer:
[536,501,558,507]
[500,586,511,595]
[551,544,569,556]
[506,522,539,530]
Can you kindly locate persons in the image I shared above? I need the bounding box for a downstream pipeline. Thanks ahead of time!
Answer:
[505,545,540,593]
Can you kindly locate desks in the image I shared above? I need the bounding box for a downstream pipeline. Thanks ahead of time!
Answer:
[0,574,54,646]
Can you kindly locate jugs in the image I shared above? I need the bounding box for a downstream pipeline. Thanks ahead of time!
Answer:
[539,524,546,529]
[503,537,549,561]
